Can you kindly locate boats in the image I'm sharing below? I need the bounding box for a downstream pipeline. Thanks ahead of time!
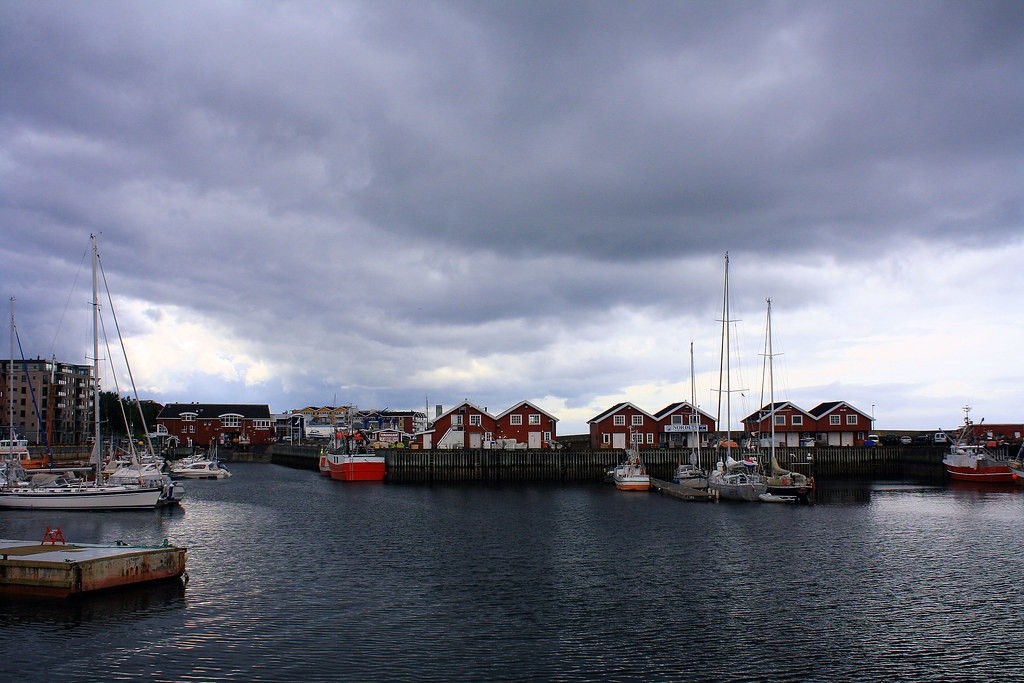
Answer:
[939,404,1018,484]
[317,393,386,482]
[613,425,651,492]
[757,493,800,504]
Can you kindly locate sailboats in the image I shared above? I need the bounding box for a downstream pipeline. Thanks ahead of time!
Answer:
[671,250,813,502]
[0,232,233,510]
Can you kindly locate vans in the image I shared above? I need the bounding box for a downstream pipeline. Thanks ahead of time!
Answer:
[869,432,949,447]
